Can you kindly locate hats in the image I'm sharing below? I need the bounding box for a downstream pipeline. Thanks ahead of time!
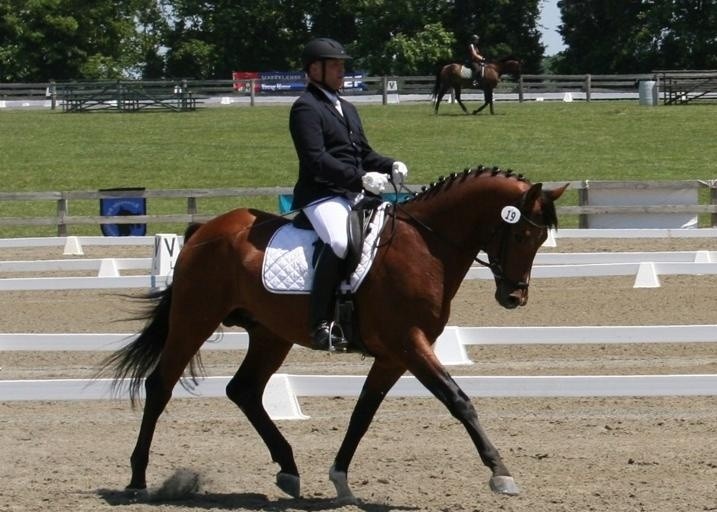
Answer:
[301,37,353,72]
[470,34,480,40]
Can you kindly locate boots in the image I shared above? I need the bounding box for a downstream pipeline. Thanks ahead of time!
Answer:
[308,242,349,350]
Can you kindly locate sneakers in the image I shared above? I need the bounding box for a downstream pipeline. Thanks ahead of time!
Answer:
[472,80,480,87]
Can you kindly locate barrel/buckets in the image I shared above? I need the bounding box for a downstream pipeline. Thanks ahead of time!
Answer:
[638,79,656,105]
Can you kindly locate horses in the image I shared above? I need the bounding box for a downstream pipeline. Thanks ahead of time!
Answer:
[90,164,571,503]
[432,56,521,115]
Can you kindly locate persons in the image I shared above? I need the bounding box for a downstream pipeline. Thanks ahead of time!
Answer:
[289,37,408,351]
[466,33,488,87]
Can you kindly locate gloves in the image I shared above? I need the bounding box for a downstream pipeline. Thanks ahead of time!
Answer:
[391,161,408,185]
[362,171,389,196]
